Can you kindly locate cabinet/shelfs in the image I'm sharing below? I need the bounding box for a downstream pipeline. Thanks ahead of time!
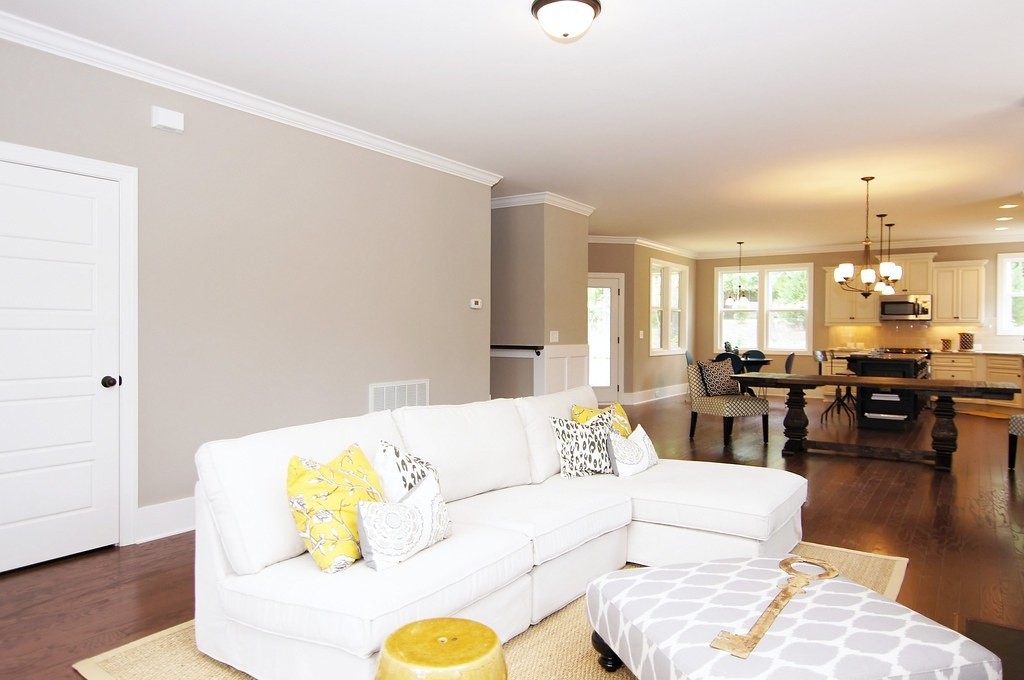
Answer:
[875,251,937,296]
[822,264,881,327]
[931,259,988,323]
[928,354,1023,419]
[846,350,931,432]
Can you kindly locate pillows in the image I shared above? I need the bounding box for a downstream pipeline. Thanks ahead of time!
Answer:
[549,402,660,478]
[285,440,453,574]
[696,358,740,397]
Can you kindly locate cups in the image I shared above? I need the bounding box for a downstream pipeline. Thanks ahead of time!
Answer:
[847,343,854,349]
[871,346,878,357]
[856,343,864,350]
[879,344,885,354]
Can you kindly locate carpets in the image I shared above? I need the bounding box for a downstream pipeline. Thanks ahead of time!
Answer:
[70,538,909,680]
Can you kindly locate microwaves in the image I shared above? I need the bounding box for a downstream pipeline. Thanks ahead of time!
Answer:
[878,295,932,321]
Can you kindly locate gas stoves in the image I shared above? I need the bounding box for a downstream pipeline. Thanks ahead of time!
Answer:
[877,348,930,353]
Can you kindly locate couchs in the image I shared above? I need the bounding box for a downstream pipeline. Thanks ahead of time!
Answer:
[194,387,809,680]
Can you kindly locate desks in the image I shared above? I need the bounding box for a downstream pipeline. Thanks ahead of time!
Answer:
[729,372,1022,470]
[708,358,773,398]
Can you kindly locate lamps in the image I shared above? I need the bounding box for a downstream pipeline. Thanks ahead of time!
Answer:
[724,241,749,309]
[833,175,902,299]
[531,0,602,41]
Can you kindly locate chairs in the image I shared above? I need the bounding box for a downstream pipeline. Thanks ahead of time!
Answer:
[687,364,769,446]
[813,349,857,426]
[1007,414,1024,469]
[685,350,796,409]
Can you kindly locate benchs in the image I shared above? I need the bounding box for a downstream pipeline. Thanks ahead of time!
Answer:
[585,554,1003,680]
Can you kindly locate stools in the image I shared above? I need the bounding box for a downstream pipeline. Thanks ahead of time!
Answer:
[373,618,508,680]
